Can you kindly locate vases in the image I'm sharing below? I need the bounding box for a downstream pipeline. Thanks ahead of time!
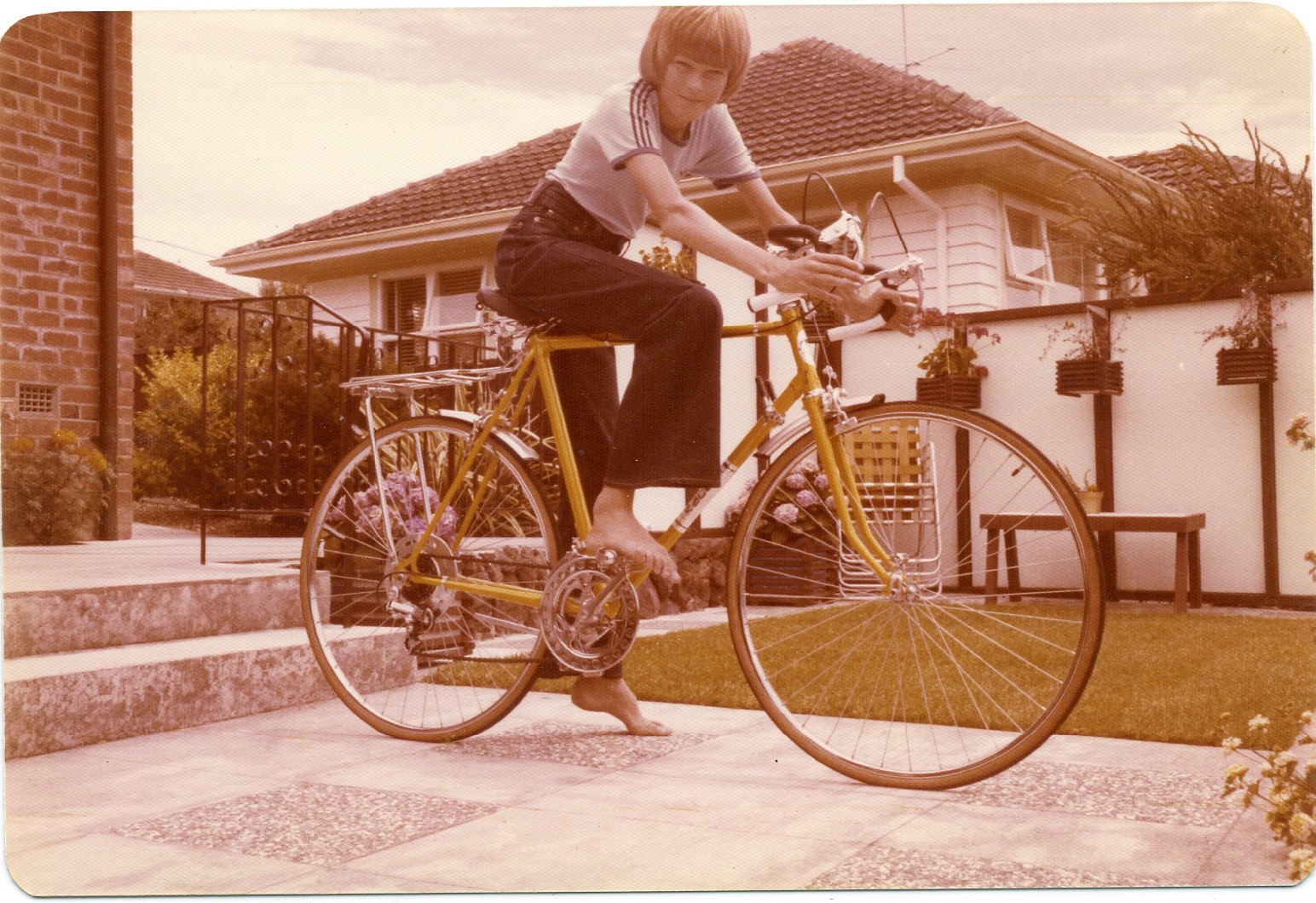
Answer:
[916,375,982,410]
[744,526,839,606]
[324,532,476,665]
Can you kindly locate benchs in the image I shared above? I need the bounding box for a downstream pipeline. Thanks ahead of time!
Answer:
[981,509,1207,617]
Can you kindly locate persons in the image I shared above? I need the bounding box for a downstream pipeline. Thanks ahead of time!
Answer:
[494,5,918,735]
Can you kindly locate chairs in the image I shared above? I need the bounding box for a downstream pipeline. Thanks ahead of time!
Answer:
[835,416,943,601]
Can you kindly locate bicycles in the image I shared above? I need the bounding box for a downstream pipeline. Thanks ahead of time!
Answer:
[299,171,1106,791]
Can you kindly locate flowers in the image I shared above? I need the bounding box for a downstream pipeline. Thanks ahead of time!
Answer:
[913,305,1001,381]
[726,460,851,546]
[301,467,458,544]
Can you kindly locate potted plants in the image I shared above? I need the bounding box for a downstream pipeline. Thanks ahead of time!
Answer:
[1053,459,1104,514]
[1045,296,1132,397]
[1198,291,1289,385]
